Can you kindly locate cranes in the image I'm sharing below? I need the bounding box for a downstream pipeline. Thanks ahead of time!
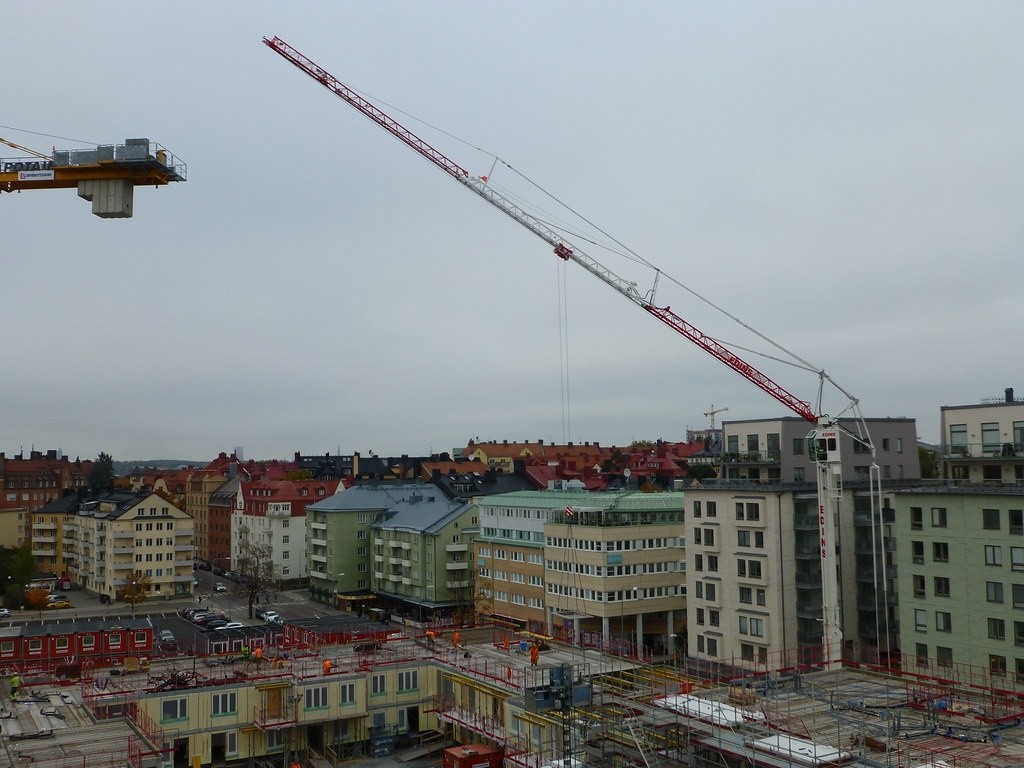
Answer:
[0,118,193,219]
[260,34,895,676]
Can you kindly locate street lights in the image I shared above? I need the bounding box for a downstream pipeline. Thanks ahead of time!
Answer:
[210,556,232,611]
[621,585,639,659]
[419,584,435,639]
[328,555,335,613]
[669,633,685,675]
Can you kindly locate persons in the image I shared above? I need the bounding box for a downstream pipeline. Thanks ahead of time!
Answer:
[265,595,269,603]
[451,630,459,647]
[426,631,437,647]
[255,595,259,605]
[243,645,249,665]
[198,595,202,604]
[10,672,19,698]
[254,645,263,671]
[290,760,300,768]
[322,658,331,673]
[530,641,539,666]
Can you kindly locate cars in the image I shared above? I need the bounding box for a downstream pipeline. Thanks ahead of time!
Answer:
[45,594,70,609]
[155,561,288,651]
[0,608,11,618]
